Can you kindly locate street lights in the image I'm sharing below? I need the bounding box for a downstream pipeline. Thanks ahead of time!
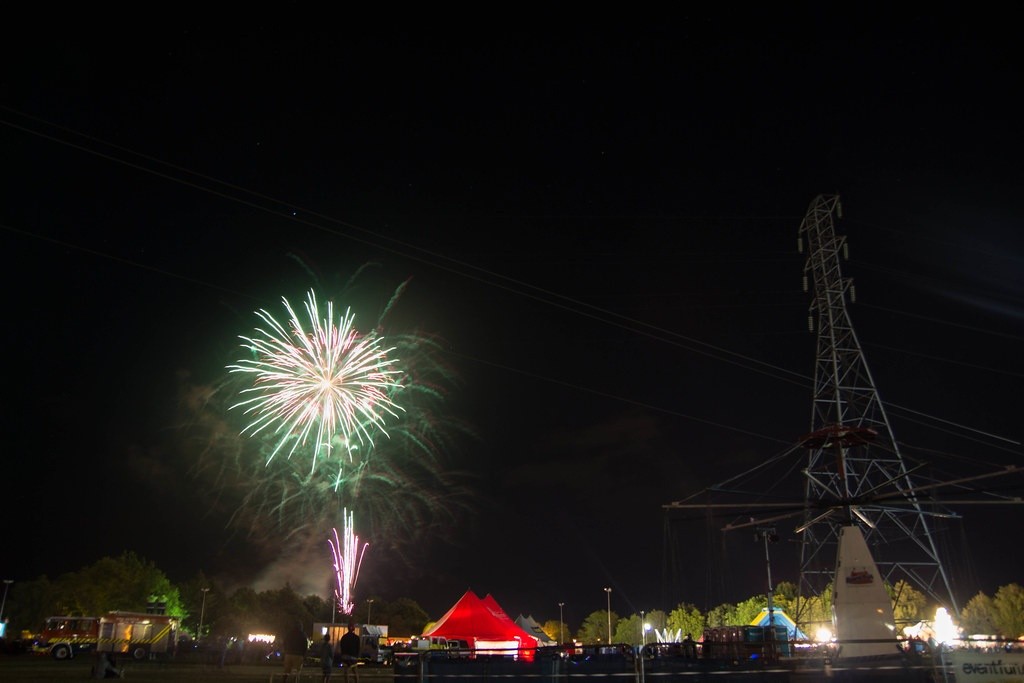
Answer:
[558,602,565,644]
[199,588,210,638]
[604,587,612,644]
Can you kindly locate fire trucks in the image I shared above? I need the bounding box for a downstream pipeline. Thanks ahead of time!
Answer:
[32,609,177,658]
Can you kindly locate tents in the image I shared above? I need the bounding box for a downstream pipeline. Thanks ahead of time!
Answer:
[418,587,556,665]
[751,606,808,641]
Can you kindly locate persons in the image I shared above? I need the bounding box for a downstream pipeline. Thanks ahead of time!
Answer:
[903,634,938,648]
[340,623,361,682]
[282,616,308,683]
[319,633,335,683]
[683,634,713,663]
